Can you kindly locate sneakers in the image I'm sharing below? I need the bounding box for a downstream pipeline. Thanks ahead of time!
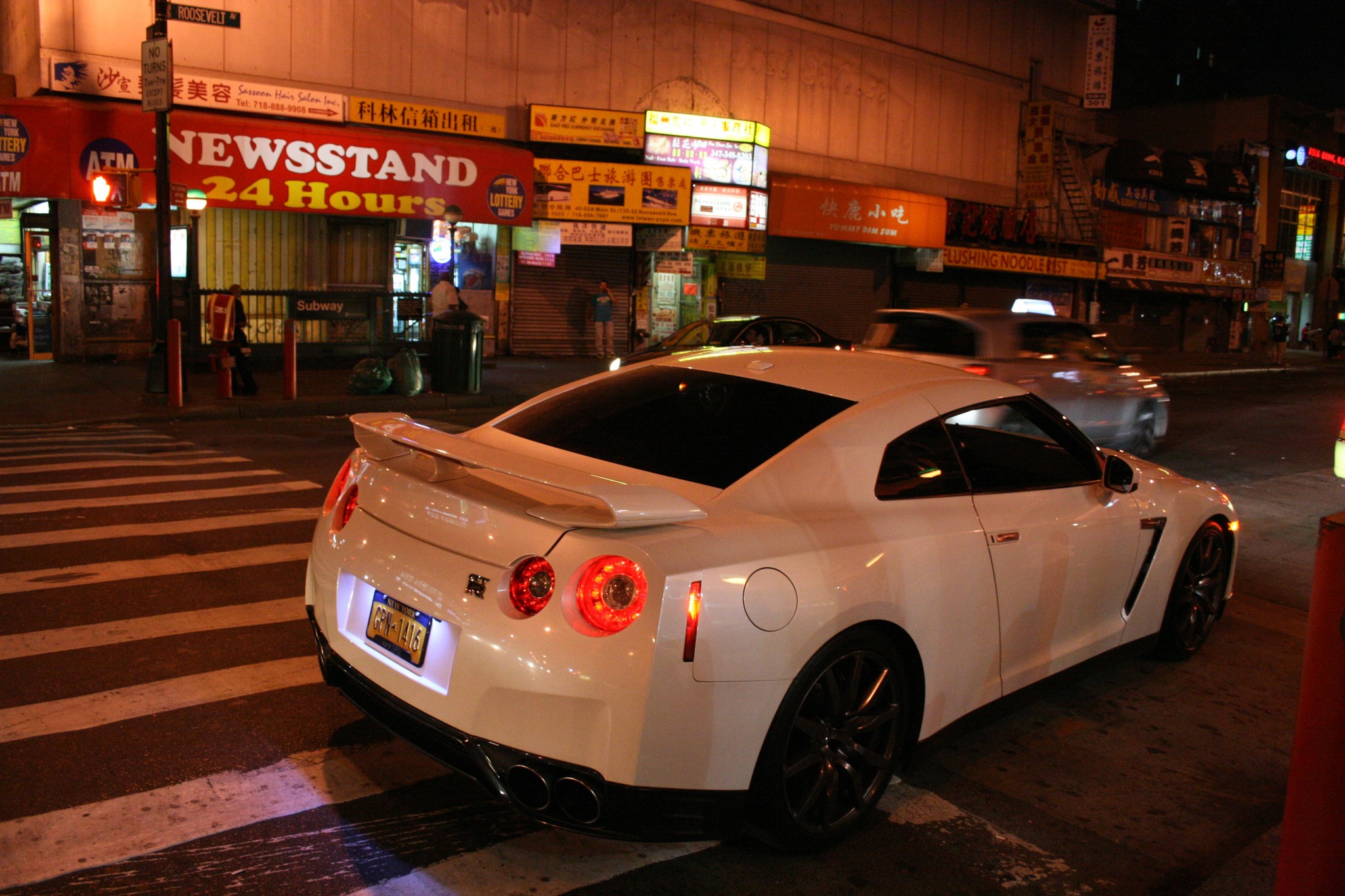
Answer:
[596,352,604,358]
[606,352,618,358]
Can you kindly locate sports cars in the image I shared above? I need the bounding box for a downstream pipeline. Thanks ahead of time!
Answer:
[301,348,1240,846]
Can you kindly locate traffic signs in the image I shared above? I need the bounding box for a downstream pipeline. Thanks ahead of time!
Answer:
[167,2,242,30]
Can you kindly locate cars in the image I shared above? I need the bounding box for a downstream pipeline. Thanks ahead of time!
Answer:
[609,315,860,373]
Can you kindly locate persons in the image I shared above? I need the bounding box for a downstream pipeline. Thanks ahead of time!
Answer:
[227,284,258,396]
[740,328,762,346]
[432,272,459,330]
[959,301,968,308]
[1271,315,1290,366]
[455,287,472,313]
[587,280,619,359]
[1301,322,1317,351]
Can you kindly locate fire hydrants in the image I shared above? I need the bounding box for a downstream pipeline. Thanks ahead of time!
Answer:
[214,354,238,399]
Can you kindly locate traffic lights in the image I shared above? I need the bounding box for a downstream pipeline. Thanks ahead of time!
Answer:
[91,170,130,205]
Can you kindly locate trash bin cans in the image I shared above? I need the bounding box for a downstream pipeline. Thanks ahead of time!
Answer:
[431,310,486,395]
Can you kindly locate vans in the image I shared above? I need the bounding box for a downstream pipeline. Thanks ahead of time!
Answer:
[860,308,1179,452]
